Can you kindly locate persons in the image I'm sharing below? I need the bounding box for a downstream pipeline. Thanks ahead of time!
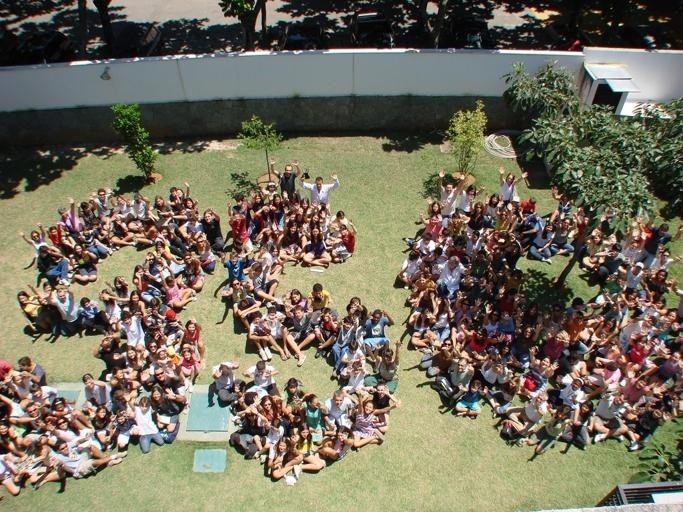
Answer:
[0,158,401,496]
[397,164,682,455]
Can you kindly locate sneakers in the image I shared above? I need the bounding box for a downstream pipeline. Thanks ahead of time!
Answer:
[314,348,328,358]
[259,349,306,366]
[184,379,194,392]
[108,451,128,466]
[593,433,640,452]
[293,464,302,479]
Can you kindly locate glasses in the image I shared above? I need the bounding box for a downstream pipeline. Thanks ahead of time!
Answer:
[149,345,157,348]
[28,408,68,451]
[155,370,164,376]
